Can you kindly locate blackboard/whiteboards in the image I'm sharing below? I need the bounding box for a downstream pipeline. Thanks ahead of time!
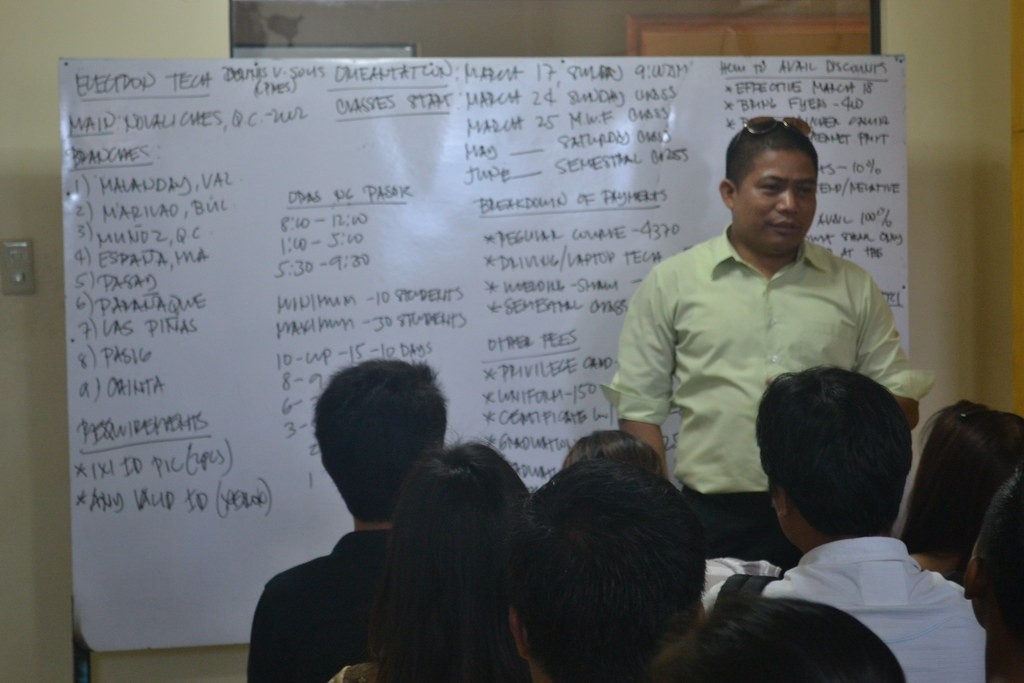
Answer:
[53,48,910,661]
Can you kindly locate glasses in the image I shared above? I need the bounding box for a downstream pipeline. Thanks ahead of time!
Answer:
[732,115,814,149]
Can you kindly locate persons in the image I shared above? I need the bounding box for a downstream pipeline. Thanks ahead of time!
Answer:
[601,120,933,564]
[248,358,1023,682]
